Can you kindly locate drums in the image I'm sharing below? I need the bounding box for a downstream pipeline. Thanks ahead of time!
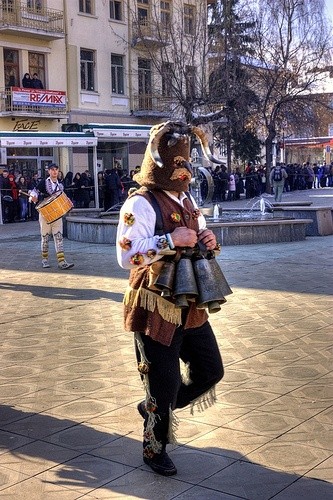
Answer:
[35,190,75,225]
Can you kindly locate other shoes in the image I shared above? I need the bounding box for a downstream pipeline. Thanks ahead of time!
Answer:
[58,261,74,269]
[42,260,50,268]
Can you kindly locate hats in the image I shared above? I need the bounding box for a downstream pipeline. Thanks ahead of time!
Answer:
[48,163,58,169]
[8,174,14,178]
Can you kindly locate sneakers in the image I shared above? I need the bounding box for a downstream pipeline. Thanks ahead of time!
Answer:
[143,453,177,476]
[138,400,149,431]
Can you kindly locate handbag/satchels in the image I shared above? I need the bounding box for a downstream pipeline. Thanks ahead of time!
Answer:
[262,176,267,183]
[2,195,13,201]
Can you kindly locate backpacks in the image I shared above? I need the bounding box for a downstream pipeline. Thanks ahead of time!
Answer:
[273,168,282,182]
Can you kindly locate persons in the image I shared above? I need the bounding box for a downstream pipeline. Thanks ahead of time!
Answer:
[29,163,75,270]
[269,162,288,203]
[270,162,333,195]
[199,161,267,204]
[98,165,141,209]
[115,119,224,478]
[0,166,95,224]
[22,72,43,111]
